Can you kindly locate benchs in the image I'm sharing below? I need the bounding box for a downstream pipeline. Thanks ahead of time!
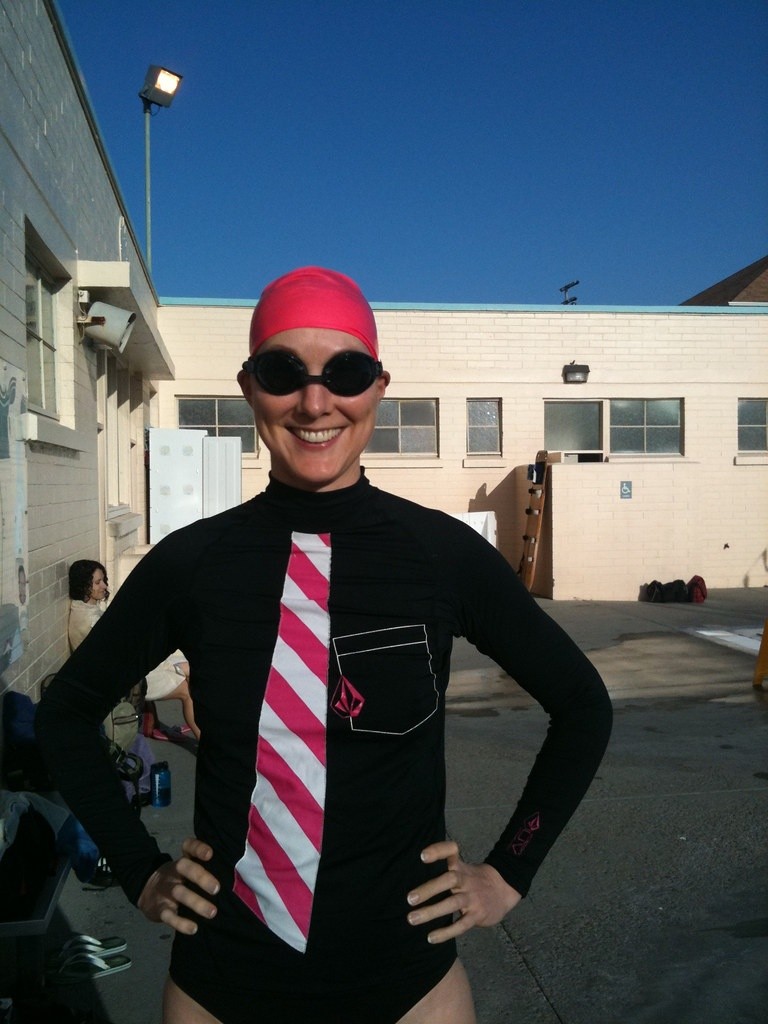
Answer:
[0,814,77,1024]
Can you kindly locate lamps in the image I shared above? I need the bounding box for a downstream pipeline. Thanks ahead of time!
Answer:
[78,303,138,356]
[562,360,590,384]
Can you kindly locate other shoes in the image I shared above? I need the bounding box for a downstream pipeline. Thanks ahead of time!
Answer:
[150,729,169,739]
[75,858,118,889]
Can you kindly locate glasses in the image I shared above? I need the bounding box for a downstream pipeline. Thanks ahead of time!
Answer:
[242,351,383,397]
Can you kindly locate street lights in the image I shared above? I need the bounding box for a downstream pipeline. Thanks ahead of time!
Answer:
[139,63,184,279]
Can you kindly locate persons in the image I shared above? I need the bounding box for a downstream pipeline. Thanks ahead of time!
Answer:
[39,265,614,1024]
[69,560,201,744]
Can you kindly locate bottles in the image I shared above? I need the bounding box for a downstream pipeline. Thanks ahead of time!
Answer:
[151,761,172,808]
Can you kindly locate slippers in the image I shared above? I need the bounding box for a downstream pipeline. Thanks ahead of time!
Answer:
[48,935,128,966]
[50,953,134,983]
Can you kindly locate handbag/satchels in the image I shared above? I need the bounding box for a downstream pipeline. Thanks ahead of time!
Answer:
[102,696,138,752]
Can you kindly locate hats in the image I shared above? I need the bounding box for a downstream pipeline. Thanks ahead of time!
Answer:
[250,267,377,361]
